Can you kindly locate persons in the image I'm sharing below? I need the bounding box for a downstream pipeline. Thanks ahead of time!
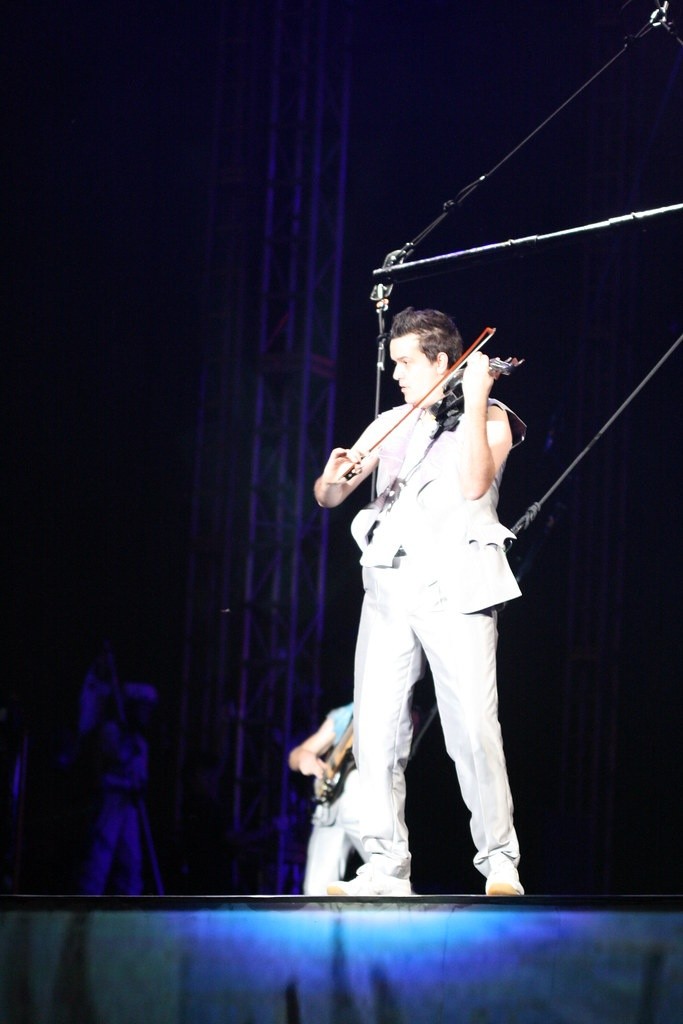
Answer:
[80,681,159,895]
[313,307,526,896]
[289,702,365,895]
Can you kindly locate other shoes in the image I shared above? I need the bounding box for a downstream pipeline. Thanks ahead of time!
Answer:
[485,866,524,896]
[326,867,412,897]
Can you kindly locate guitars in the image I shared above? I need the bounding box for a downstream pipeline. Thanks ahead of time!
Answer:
[310,712,358,806]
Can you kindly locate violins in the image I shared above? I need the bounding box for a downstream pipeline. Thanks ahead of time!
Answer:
[430,353,529,442]
